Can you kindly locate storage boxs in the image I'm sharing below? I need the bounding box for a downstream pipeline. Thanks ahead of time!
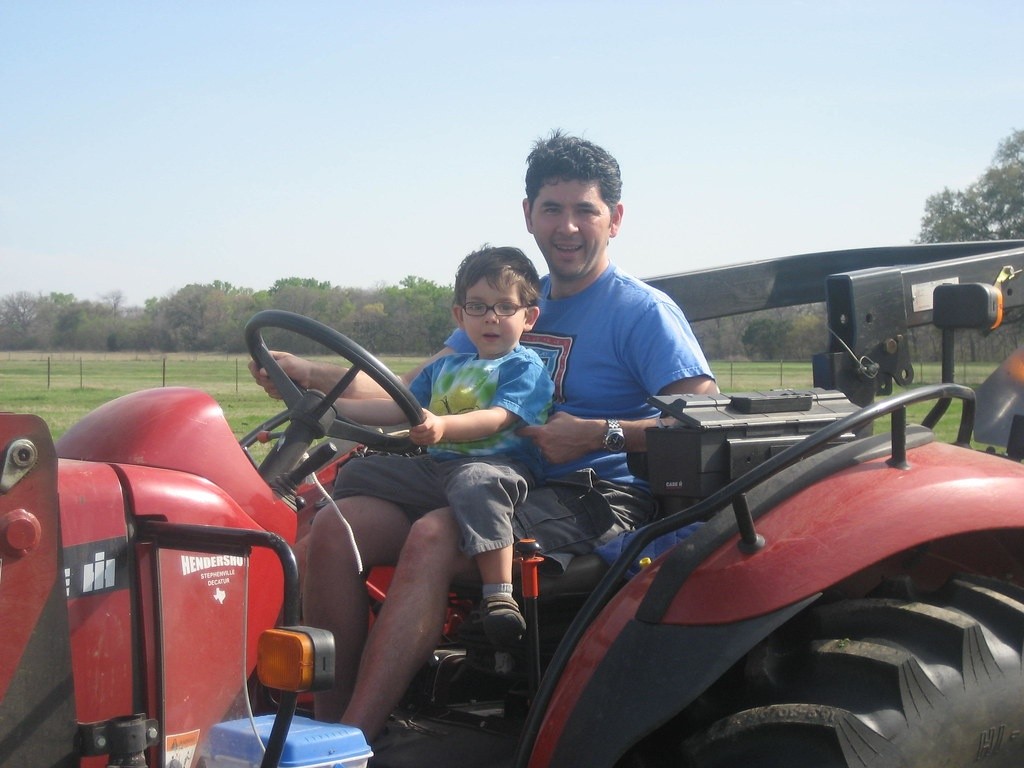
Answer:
[202,715,374,768]
[643,386,874,497]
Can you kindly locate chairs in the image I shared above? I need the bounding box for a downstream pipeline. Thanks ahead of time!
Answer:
[503,495,678,609]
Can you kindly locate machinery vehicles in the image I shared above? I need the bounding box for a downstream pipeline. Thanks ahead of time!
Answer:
[0,237,1024,768]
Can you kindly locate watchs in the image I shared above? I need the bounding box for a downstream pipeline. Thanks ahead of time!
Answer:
[602,418,626,454]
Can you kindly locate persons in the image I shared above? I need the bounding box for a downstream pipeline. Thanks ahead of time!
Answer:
[335,246,556,645]
[247,130,720,744]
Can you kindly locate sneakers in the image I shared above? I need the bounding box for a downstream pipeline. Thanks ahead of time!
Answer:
[482,596,526,647]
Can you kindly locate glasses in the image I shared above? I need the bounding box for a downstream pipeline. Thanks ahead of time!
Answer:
[460,302,531,316]
